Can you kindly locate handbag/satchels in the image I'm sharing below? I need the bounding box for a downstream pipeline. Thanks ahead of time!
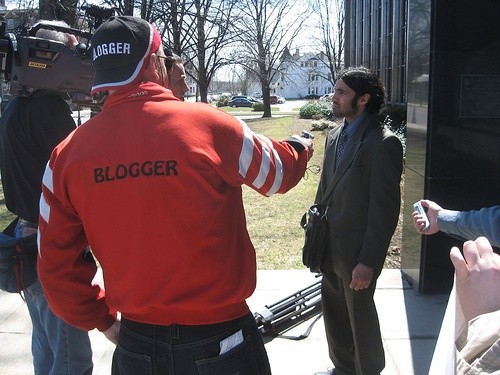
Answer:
[300,204,329,274]
[0,216,38,293]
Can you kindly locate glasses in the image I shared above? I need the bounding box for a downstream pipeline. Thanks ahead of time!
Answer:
[146,56,174,74]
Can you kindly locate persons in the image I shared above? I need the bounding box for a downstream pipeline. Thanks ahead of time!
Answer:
[450,236,500,375]
[411,198,500,247]
[311,65,403,375]
[37,14,316,375]
[0,21,188,375]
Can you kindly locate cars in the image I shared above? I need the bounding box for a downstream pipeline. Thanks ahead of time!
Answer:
[319,94,334,101]
[227,92,286,108]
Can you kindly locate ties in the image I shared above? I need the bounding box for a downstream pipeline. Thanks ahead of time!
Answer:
[335,128,349,171]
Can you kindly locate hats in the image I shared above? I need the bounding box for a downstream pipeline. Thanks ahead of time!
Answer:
[90,16,161,96]
